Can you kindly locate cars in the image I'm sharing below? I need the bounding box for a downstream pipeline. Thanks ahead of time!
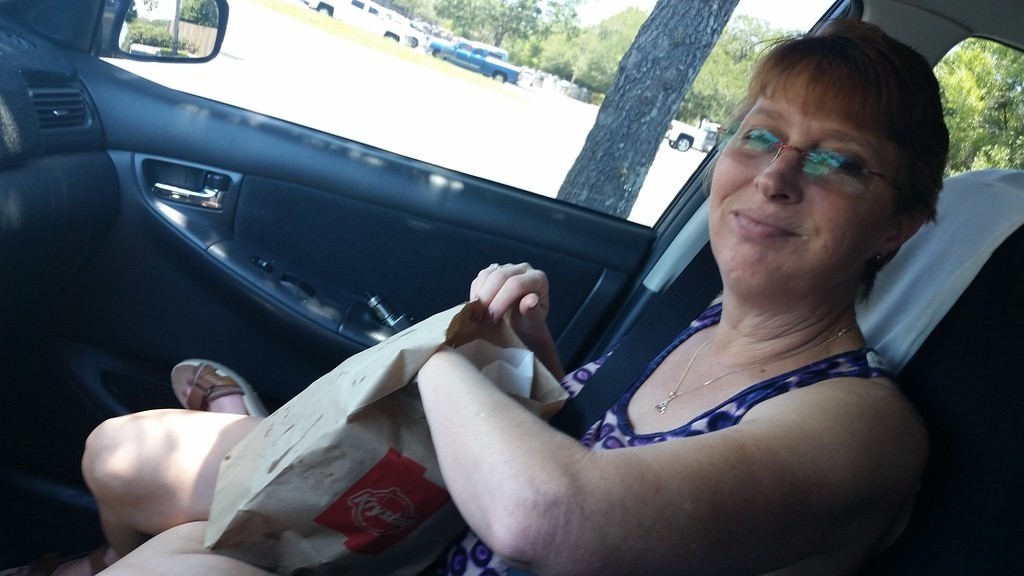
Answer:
[99,12,135,57]
[307,0,427,48]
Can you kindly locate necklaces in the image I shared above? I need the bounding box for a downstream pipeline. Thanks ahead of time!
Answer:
[654,321,858,415]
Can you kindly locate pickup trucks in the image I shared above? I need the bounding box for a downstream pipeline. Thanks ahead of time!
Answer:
[665,119,722,152]
[424,38,524,85]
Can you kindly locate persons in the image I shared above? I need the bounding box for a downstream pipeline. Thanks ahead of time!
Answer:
[0,20,948,576]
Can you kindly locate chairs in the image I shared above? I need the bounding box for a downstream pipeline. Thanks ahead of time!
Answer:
[853,161,1024,504]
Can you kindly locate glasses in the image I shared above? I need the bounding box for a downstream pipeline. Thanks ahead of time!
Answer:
[713,121,908,200]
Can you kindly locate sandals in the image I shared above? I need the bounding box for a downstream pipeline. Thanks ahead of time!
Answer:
[171,359,269,419]
[0,545,108,576]
